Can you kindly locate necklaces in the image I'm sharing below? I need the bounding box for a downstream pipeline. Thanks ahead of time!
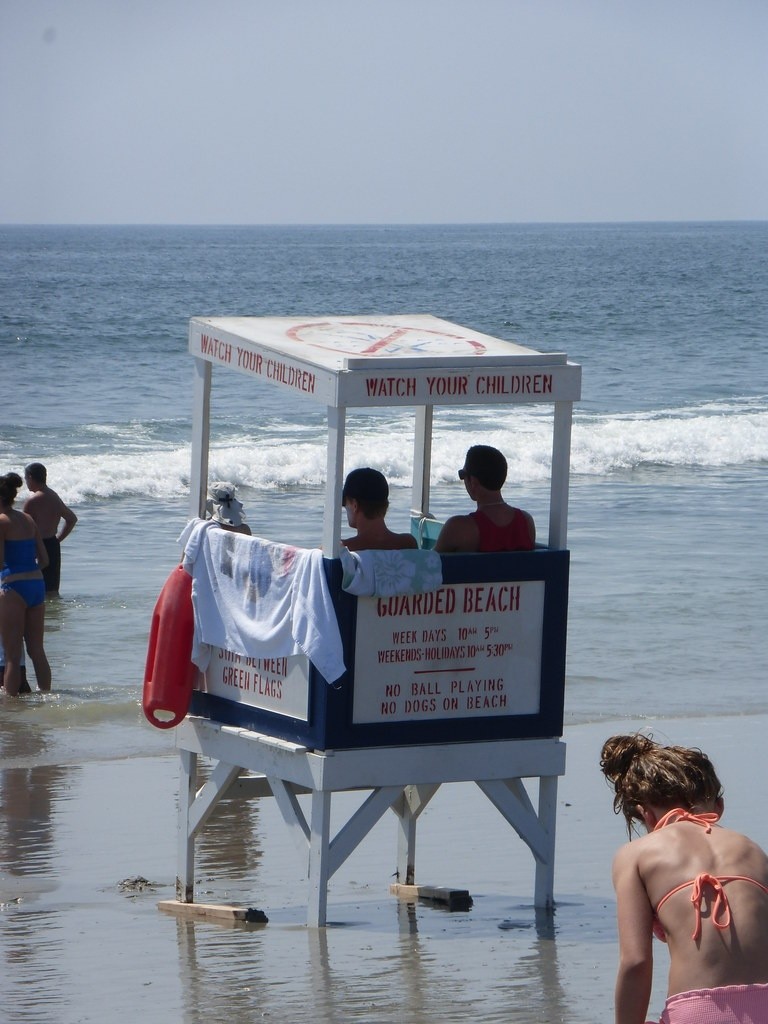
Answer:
[478,501,506,507]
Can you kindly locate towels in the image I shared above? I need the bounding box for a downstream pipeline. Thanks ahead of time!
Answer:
[336,542,445,597]
[171,516,346,686]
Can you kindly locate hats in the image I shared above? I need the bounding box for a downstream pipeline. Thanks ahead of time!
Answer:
[206,482,246,527]
[343,467,388,501]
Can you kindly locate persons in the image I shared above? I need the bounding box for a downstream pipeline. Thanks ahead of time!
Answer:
[341,467,418,551]
[207,482,252,536]
[600,728,768,1024]
[0,473,52,697]
[22,463,78,591]
[432,445,536,552]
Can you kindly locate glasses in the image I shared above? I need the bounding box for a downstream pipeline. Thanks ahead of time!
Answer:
[459,470,477,480]
